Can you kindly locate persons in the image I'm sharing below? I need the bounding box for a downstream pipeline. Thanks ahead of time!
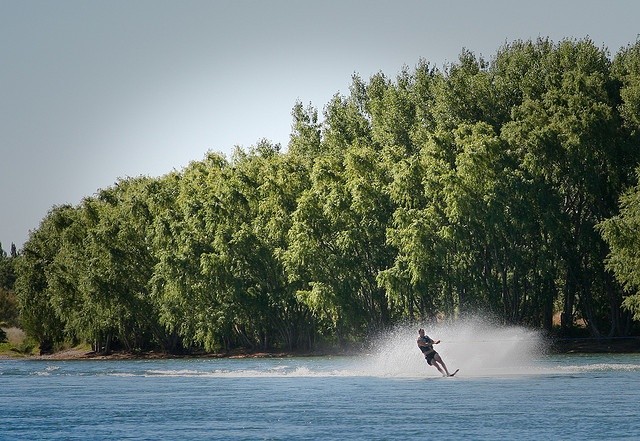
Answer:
[417,329,453,378]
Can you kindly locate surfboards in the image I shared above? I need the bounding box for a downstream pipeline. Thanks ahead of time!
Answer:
[450,369,459,378]
